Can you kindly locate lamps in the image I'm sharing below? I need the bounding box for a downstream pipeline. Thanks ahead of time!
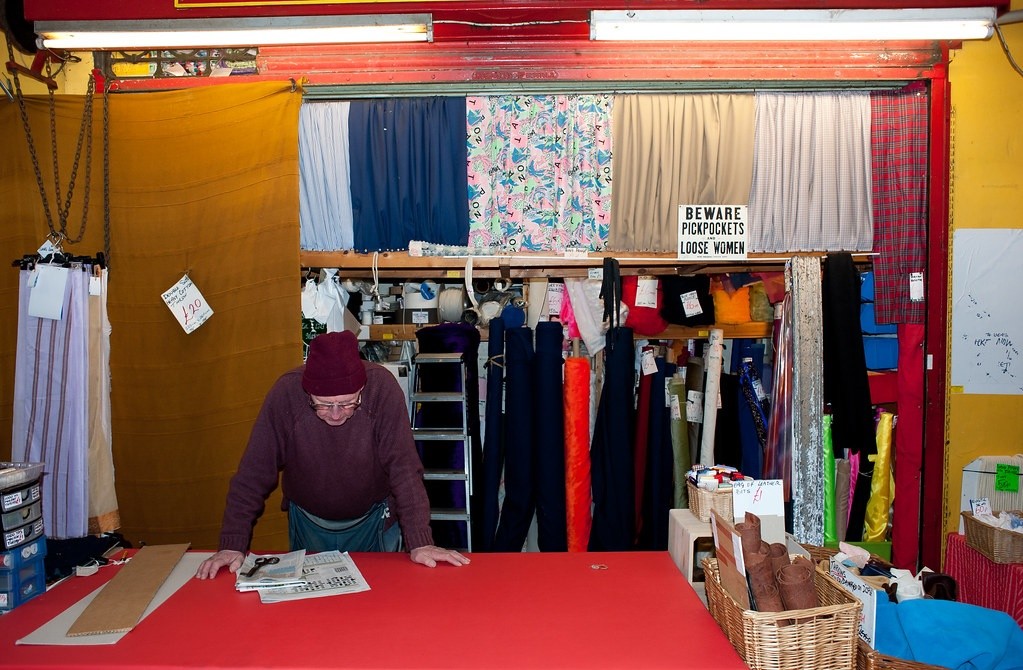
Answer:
[587,6,997,41]
[34,12,433,50]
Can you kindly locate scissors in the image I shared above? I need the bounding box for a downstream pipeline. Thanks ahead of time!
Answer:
[247,556,280,578]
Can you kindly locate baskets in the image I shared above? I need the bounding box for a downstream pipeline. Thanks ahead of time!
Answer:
[801,543,951,670]
[685,476,754,522]
[961,510,1023,564]
[703,554,863,670]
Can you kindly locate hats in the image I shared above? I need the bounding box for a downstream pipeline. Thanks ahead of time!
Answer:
[302,329,367,396]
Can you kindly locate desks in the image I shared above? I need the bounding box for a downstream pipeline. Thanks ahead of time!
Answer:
[0,550,751,670]
[942,532,1023,632]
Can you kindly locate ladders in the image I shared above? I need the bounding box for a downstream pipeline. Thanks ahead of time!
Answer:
[405,351,474,554]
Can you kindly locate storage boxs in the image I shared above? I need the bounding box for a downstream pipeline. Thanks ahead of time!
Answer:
[0,461,49,610]
[825,540,892,563]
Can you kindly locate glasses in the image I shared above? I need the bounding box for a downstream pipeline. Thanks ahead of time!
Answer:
[308,395,362,411]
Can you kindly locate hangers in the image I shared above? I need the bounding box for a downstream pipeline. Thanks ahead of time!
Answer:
[11,230,106,275]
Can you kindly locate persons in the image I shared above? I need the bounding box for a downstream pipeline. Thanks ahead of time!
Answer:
[196,331,470,579]
